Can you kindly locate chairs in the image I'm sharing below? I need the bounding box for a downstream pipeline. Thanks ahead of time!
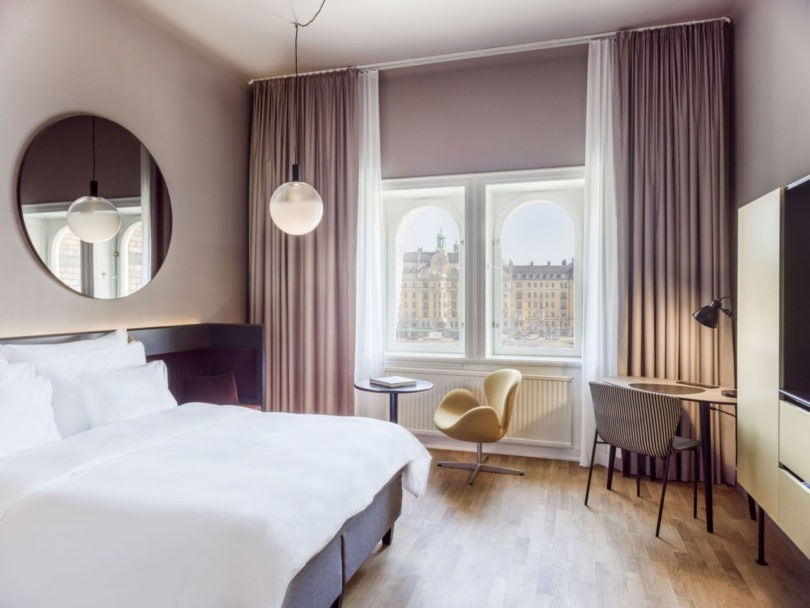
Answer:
[583,381,703,537]
[434,368,525,485]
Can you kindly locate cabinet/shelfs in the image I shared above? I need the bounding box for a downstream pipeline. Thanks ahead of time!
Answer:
[736,184,810,565]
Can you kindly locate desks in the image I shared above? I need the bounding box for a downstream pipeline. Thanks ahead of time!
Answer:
[353,379,433,423]
[604,375,756,533]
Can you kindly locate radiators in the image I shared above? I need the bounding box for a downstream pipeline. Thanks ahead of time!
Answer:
[384,366,574,450]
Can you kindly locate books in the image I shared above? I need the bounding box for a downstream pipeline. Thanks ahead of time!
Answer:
[370,375,416,388]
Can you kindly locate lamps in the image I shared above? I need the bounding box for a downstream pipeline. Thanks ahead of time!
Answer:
[269,22,323,237]
[68,117,121,243]
[691,294,736,398]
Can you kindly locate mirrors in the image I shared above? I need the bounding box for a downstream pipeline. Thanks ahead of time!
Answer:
[17,114,173,299]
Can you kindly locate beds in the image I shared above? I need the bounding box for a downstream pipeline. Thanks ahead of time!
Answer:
[0,322,432,608]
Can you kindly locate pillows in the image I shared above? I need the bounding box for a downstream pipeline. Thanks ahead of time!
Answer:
[0,327,178,459]
[187,370,241,406]
